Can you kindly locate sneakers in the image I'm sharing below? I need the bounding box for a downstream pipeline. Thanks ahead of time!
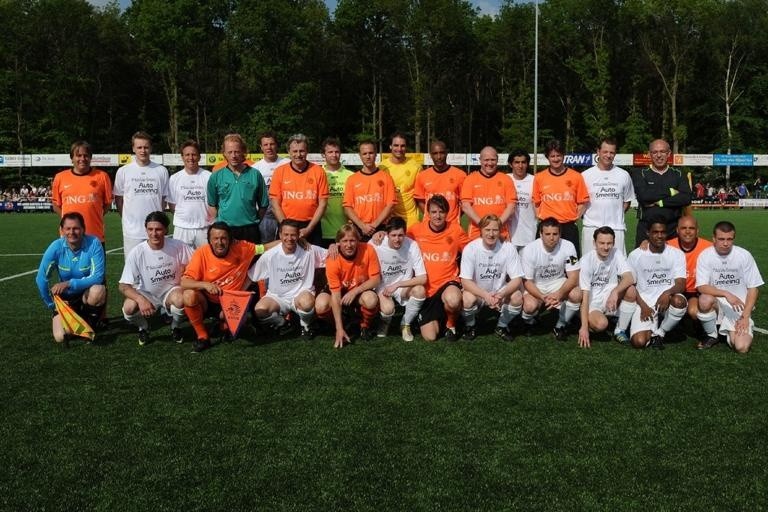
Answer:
[170,326,184,344]
[137,320,151,347]
[190,337,209,354]
[611,331,632,347]
[357,326,568,343]
[217,327,233,345]
[650,334,665,351]
[297,322,313,340]
[695,336,721,351]
[265,320,292,343]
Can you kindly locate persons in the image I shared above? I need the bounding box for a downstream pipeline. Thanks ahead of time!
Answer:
[695,221,765,352]
[34,211,106,343]
[52,140,113,251]
[691,173,767,211]
[112,129,710,347]
[1,182,53,214]
